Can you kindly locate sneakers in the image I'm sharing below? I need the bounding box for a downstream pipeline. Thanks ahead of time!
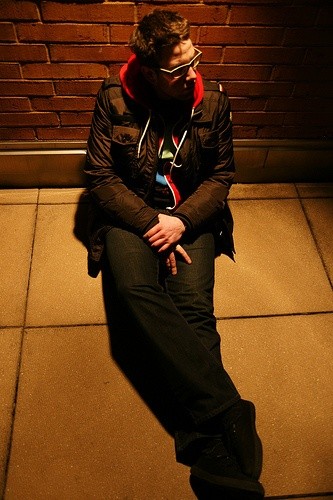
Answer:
[189,399,265,499]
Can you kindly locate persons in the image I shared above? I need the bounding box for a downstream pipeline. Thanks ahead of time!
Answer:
[81,9,263,500]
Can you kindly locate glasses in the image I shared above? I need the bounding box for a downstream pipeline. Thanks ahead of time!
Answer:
[150,47,203,79]
